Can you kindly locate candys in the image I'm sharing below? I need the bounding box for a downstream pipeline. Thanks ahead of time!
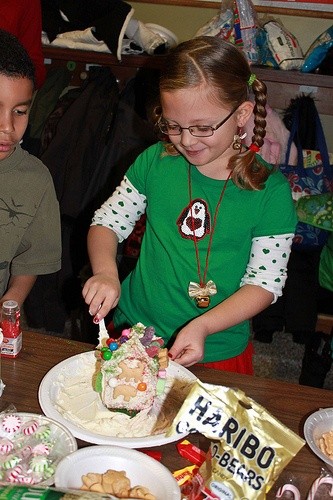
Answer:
[1,415,57,483]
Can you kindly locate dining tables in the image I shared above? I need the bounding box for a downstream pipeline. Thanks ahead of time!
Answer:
[0,327,333,500]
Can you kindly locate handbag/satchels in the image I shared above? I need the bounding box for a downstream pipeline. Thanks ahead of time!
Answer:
[29,65,161,235]
[271,108,333,248]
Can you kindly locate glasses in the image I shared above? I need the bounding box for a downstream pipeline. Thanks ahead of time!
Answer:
[156,101,240,138]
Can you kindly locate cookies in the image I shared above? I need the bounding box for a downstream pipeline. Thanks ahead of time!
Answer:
[318,431,333,461]
[80,470,156,500]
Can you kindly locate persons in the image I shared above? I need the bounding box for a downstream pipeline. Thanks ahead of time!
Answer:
[0,30,62,312]
[82,35,298,376]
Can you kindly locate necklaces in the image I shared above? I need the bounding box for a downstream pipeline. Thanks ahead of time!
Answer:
[188,143,242,309]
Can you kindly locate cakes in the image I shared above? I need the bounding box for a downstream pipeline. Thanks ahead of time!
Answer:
[98,322,168,410]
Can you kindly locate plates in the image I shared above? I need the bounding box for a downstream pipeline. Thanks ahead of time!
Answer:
[55,445,181,500]
[38,349,204,449]
[304,407,332,473]
[0,412,77,488]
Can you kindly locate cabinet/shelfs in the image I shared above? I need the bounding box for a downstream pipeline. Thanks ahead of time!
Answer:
[41,0,333,116]
